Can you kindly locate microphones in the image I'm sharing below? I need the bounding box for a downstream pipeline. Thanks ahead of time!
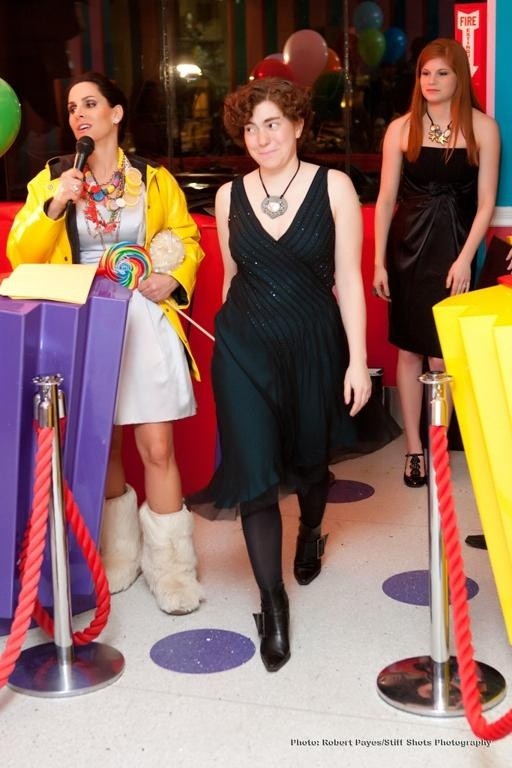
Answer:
[66,135,96,206]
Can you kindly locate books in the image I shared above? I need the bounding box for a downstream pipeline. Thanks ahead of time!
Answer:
[0,263,99,305]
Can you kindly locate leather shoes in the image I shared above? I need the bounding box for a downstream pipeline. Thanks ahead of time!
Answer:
[404,453,427,489]
[292,517,329,587]
[252,587,290,674]
[466,534,487,549]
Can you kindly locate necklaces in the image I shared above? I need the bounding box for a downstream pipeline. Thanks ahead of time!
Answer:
[424,105,462,144]
[76,148,142,253]
[255,161,302,219]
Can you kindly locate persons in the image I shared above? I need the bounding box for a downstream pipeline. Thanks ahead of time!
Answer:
[184,74,404,673]
[372,37,504,490]
[3,76,203,615]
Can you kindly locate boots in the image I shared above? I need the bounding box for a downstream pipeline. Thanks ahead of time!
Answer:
[137,499,202,617]
[97,483,141,595]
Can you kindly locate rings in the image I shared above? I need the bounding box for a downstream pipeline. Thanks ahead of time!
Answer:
[73,186,79,193]
[460,283,469,291]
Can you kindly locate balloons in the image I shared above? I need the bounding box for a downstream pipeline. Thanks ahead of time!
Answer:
[1,77,21,154]
[358,31,385,69]
[249,29,342,90]
[354,2,383,32]
[384,28,407,64]
